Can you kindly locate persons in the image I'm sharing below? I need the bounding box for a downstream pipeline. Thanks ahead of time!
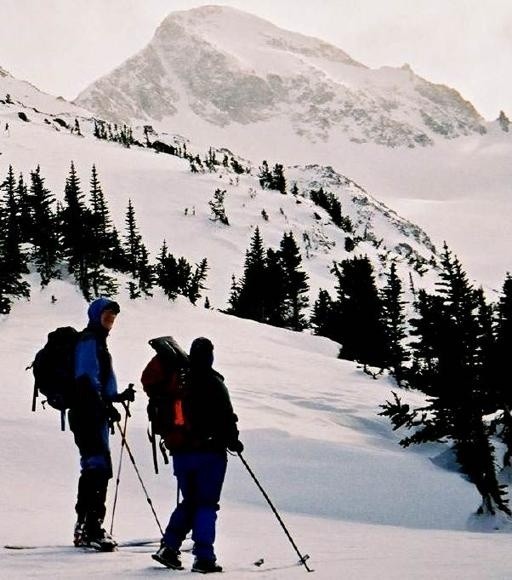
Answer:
[63,299,136,553]
[151,336,243,572]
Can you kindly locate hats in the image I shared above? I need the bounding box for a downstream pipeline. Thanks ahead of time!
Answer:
[191,338,214,356]
[89,299,120,320]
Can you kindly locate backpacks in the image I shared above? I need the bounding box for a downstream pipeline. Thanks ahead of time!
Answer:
[33,326,90,411]
[140,352,206,450]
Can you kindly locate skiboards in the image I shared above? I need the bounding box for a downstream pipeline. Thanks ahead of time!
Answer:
[120,554,309,577]
[4,533,195,556]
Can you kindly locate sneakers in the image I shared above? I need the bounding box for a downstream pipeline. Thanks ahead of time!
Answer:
[152,544,182,567]
[73,522,119,552]
[192,557,222,574]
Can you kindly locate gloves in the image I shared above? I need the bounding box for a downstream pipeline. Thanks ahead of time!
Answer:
[122,388,135,402]
[107,406,121,428]
[229,440,243,453]
